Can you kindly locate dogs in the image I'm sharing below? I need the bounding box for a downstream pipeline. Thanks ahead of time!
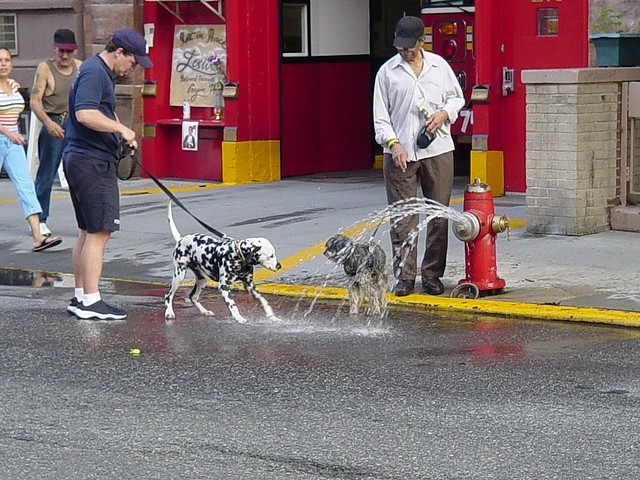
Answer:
[164,199,281,324]
[323,234,387,318]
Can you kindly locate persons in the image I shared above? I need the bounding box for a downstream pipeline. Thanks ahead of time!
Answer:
[373,15,465,296]
[62,30,153,321]
[1,49,64,252]
[30,29,82,235]
[183,126,195,149]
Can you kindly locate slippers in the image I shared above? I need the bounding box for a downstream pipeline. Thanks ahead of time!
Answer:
[33,237,61,252]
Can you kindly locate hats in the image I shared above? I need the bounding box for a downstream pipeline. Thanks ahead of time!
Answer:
[113,28,154,69]
[54,29,78,50]
[393,16,425,48]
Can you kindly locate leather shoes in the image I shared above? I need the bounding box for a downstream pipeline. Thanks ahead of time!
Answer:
[395,280,414,295]
[423,276,444,295]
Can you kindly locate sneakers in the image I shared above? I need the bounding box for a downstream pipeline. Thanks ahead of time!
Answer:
[28,222,50,236]
[75,302,127,320]
[66,297,82,313]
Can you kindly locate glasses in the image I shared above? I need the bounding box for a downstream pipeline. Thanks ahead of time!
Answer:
[394,46,416,51]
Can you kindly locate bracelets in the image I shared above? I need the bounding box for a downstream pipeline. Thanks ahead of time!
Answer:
[385,139,400,149]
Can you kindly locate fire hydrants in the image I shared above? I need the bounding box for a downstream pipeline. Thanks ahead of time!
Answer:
[448,178,511,301]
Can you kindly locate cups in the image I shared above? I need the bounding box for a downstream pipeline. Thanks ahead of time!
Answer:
[182,99,190,120]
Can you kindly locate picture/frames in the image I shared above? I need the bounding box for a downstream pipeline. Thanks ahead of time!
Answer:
[180,120,200,153]
